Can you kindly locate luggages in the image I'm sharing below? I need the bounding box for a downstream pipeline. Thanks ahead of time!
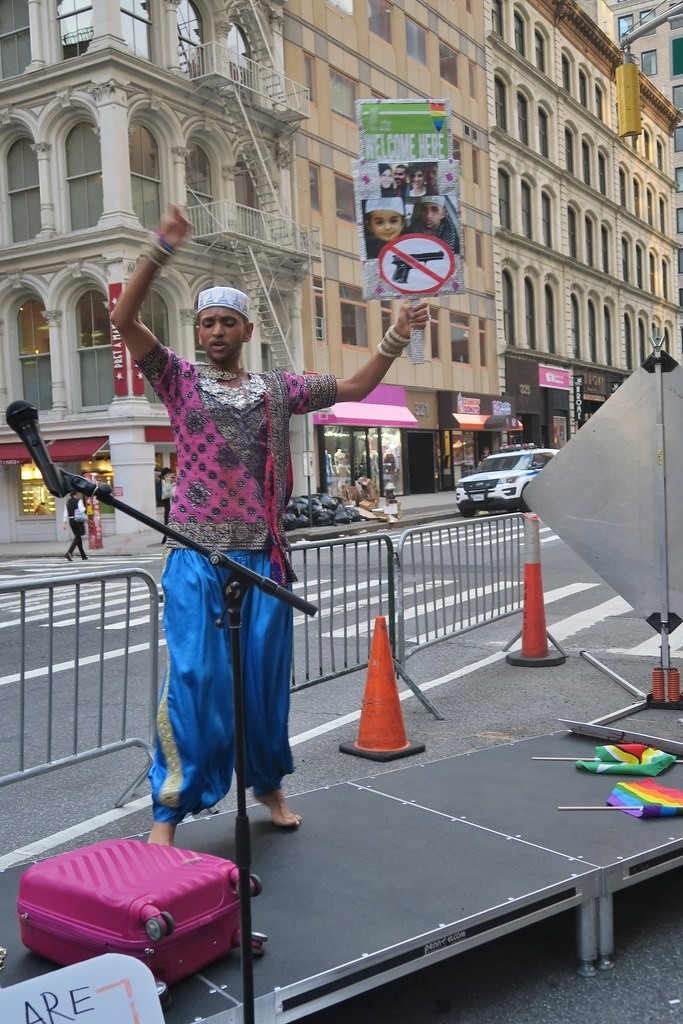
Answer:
[16,837,268,1012]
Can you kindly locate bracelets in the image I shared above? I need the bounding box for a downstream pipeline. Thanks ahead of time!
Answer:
[388,324,411,343]
[144,238,175,268]
[377,339,403,359]
[384,329,408,347]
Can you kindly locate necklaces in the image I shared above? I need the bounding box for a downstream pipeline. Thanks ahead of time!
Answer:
[193,359,245,381]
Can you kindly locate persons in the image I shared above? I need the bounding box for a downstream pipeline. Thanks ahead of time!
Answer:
[478,447,492,467]
[159,466,177,545]
[65,492,89,562]
[360,162,461,256]
[324,448,399,496]
[107,199,430,846]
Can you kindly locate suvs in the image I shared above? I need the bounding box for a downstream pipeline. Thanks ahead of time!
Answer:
[456,443,560,517]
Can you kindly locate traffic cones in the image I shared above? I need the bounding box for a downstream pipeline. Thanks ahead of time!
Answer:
[338,616,425,762]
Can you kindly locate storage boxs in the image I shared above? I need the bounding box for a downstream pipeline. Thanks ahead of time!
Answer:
[343,477,380,513]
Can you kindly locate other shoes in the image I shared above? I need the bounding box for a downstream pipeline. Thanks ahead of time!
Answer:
[64,553,72,561]
[82,556,88,559]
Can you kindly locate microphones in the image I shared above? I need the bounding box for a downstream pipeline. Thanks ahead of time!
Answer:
[5,400,62,498]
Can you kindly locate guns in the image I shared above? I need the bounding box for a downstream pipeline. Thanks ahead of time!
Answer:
[391,249,445,284]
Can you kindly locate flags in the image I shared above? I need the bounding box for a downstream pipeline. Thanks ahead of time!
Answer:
[575,744,678,776]
[605,778,683,817]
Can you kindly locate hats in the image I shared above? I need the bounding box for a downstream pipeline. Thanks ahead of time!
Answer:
[197,286,251,321]
[422,196,445,208]
[365,197,405,216]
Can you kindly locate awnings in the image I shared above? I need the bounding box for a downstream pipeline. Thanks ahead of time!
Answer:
[0,435,111,465]
[454,414,523,431]
[325,400,420,428]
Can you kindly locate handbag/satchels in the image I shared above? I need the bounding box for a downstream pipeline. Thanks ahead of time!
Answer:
[74,508,88,522]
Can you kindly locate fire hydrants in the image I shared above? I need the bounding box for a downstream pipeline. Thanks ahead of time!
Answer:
[384,482,397,506]
